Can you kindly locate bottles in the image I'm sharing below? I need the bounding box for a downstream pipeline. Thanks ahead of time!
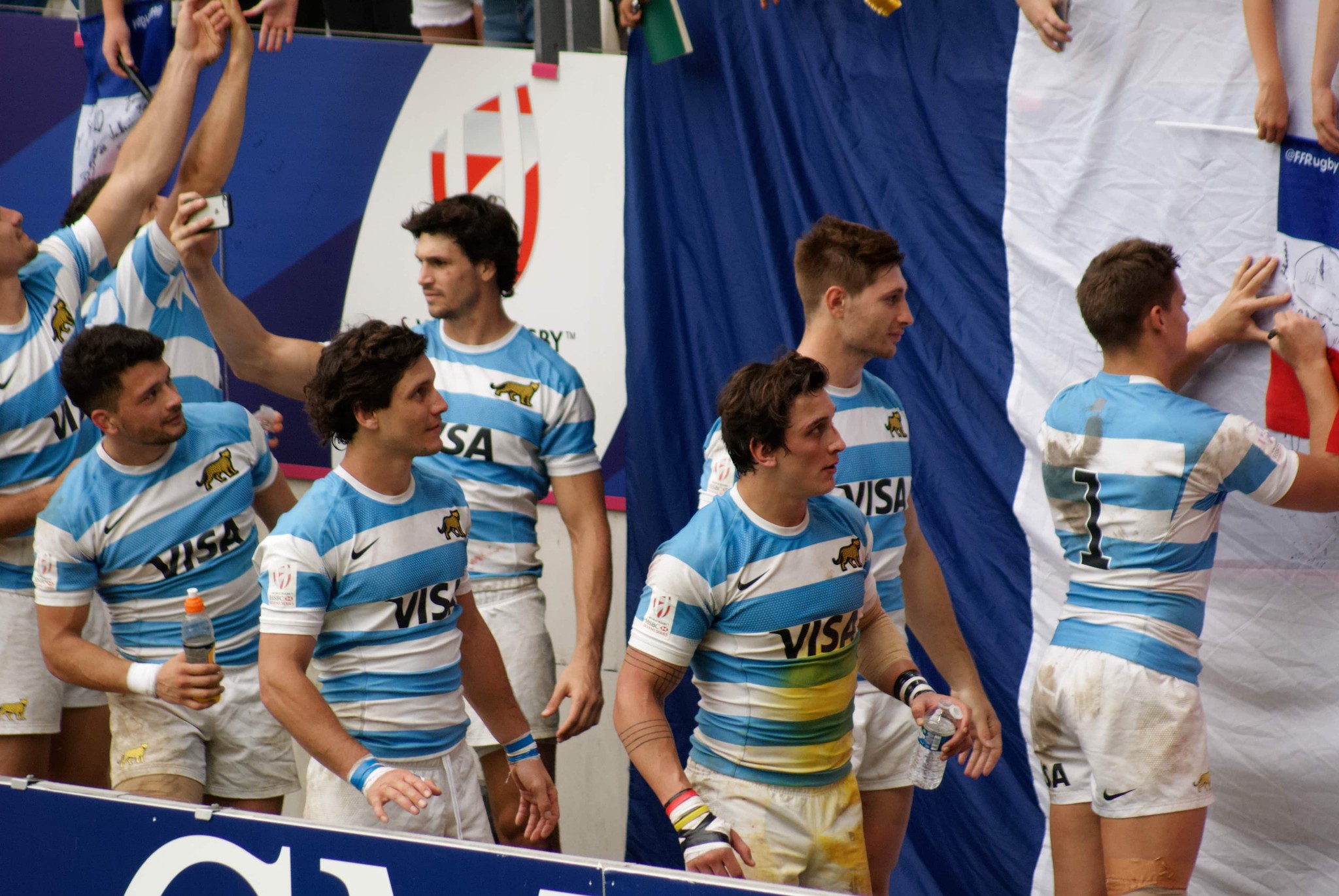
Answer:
[907,698,962,790]
[181,587,220,704]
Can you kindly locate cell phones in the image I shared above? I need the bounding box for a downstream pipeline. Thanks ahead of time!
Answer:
[184,192,233,233]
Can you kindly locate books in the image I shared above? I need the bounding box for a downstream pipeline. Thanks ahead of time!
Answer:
[638,0,694,64]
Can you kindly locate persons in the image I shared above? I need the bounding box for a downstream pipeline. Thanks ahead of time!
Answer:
[252,317,560,844]
[613,351,975,896]
[1243,0,1339,154]
[0,0,535,815]
[618,0,1072,55]
[696,213,1003,896]
[169,192,613,852]
[1028,238,1339,896]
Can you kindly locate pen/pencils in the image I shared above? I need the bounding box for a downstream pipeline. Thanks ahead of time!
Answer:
[1268,325,1278,339]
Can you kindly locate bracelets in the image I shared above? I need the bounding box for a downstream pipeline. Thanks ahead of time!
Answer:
[500,731,540,762]
[346,754,397,798]
[894,669,937,707]
[126,662,163,699]
